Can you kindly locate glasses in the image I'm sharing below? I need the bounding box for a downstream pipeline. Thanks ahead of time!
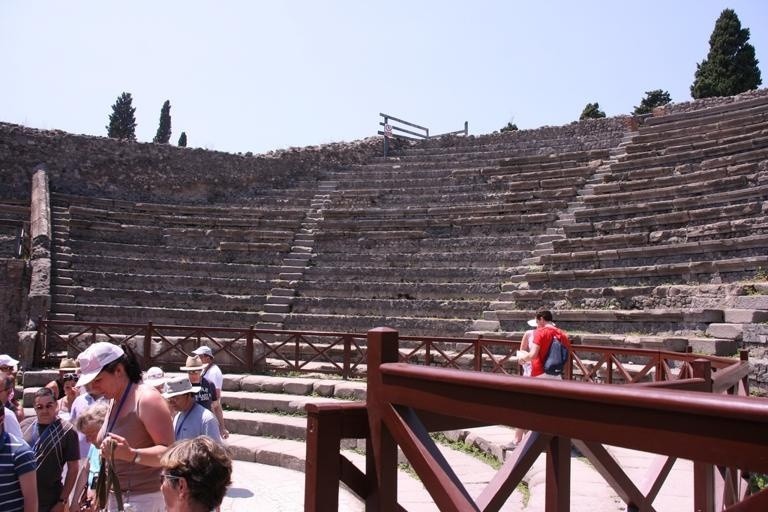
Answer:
[159,471,179,483]
[36,405,54,410]
[1,366,18,372]
[63,372,79,379]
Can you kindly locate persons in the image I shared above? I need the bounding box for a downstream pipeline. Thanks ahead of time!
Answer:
[0,341,229,512]
[516,319,555,375]
[512,311,575,448]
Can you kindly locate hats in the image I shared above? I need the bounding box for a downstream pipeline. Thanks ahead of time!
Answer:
[147,366,165,386]
[75,342,125,387]
[162,374,201,398]
[59,358,80,372]
[528,316,556,327]
[191,345,214,357]
[180,355,209,370]
[0,354,20,366]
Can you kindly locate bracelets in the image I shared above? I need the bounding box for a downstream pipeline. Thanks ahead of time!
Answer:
[520,357,526,363]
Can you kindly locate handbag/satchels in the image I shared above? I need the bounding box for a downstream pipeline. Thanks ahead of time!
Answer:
[543,336,568,374]
